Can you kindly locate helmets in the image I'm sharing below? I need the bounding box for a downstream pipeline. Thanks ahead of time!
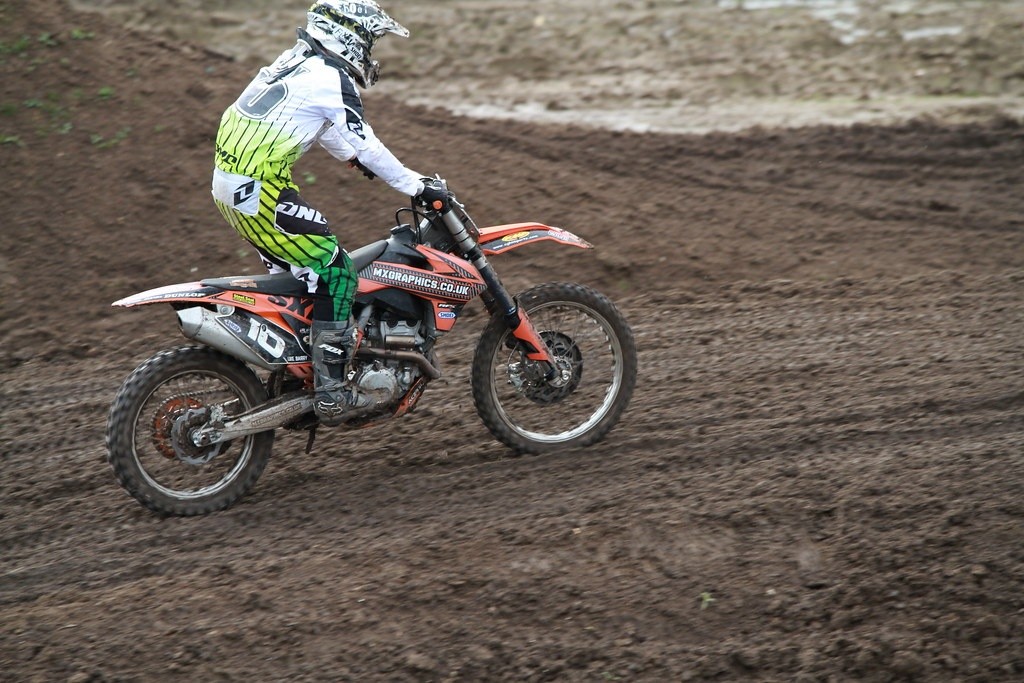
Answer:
[306,0,410,89]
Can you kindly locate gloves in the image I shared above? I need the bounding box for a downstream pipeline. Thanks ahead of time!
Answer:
[414,187,448,213]
[349,155,378,180]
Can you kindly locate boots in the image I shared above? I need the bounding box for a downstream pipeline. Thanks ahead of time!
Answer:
[311,315,391,427]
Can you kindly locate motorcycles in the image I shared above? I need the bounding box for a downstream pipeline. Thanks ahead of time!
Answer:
[104,173,638,517]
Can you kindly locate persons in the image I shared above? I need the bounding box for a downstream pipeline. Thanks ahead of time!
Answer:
[211,0,455,427]
